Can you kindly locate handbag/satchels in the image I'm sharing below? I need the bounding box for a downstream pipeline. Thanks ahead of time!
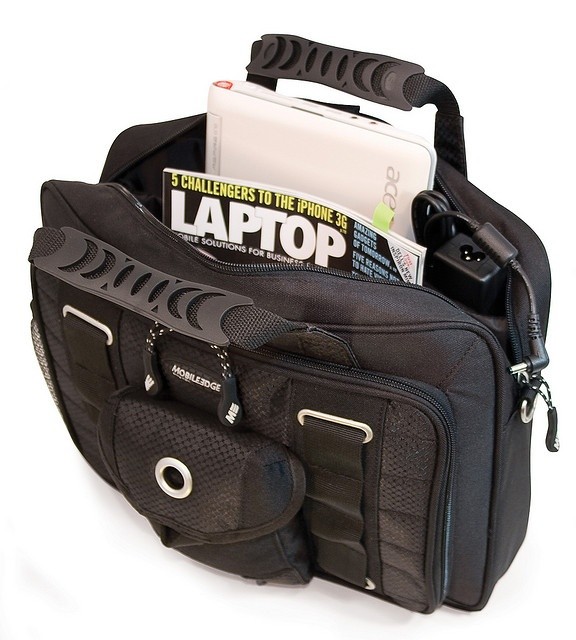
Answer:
[27,34,561,615]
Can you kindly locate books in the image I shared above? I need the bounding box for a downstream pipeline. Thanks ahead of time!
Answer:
[161,167,426,289]
